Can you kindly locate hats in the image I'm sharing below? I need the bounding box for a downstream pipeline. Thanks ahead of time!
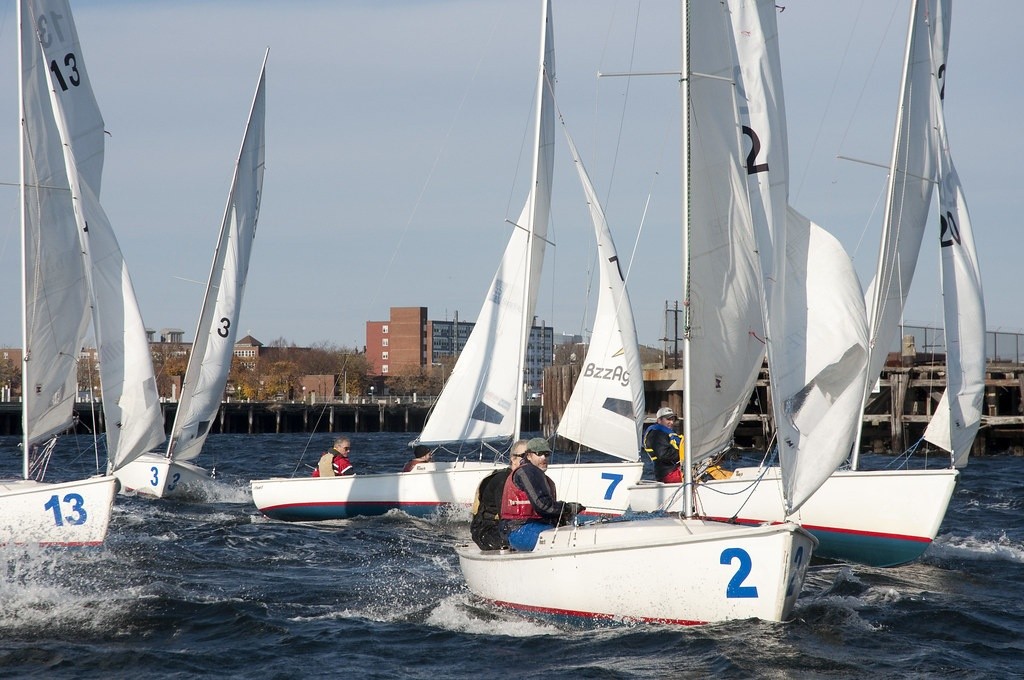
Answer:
[414,446,431,459]
[656,407,678,420]
[526,437,553,453]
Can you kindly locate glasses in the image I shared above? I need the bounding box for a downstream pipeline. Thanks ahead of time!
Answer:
[338,445,352,451]
[513,453,524,458]
[530,451,551,456]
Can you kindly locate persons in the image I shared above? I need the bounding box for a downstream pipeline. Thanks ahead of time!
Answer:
[402,445,433,472]
[643,407,682,483]
[500,438,586,551]
[470,439,528,551]
[310,435,367,477]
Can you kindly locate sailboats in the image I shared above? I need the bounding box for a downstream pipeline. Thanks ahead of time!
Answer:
[0,0,166,548]
[455,1,874,624]
[106,47,273,497]
[248,0,645,526]
[628,0,987,570]
